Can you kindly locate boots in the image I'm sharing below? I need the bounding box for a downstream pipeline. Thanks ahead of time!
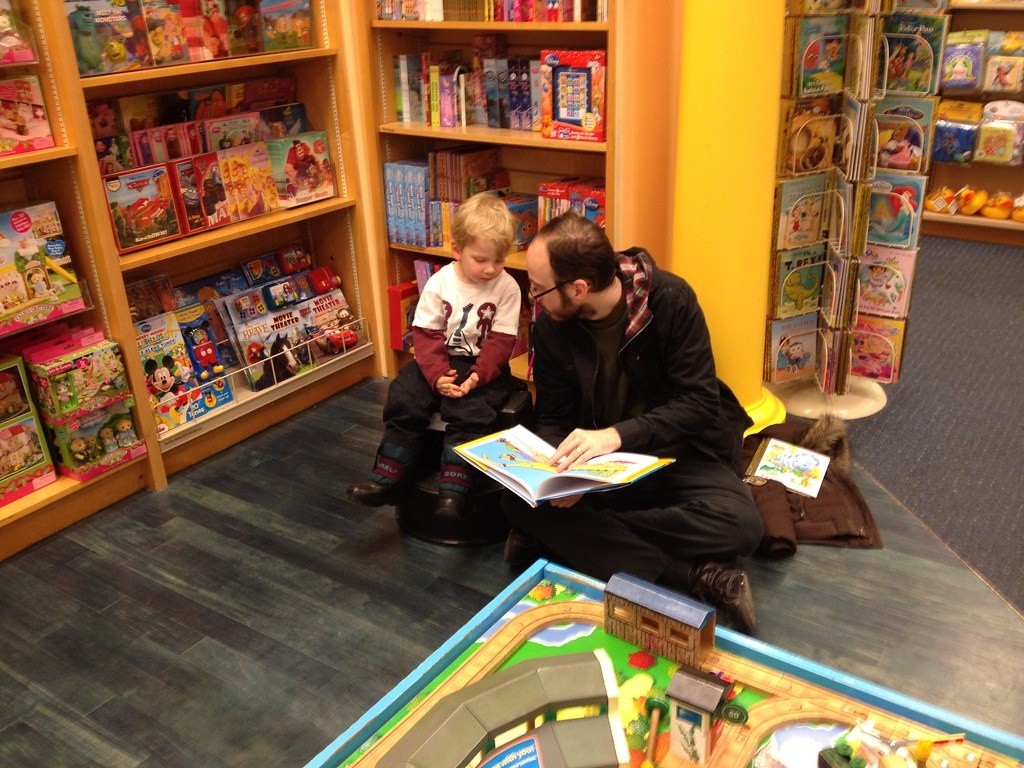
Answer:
[346,439,419,508]
[434,440,474,520]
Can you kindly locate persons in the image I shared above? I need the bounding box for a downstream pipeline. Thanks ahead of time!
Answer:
[347,191,522,535]
[502,207,765,635]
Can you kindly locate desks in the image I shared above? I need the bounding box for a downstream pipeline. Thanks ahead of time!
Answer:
[306,558,1024,768]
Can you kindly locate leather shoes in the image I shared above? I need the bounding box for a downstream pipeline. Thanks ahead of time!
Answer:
[688,560,755,631]
[504,526,535,562]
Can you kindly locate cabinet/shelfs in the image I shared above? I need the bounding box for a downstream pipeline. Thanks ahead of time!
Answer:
[0,0,145,558]
[48,0,359,481]
[343,0,686,385]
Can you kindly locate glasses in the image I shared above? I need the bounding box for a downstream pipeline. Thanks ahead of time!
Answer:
[526,279,590,306]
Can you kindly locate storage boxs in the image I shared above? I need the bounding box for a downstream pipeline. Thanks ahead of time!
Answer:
[0,74,55,157]
[0,200,84,336]
[533,176,606,240]
[0,325,148,509]
[540,47,608,145]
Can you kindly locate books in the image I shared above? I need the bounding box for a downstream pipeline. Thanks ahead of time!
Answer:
[760,1,952,388]
[391,34,543,132]
[63,0,318,80]
[745,436,831,499]
[85,73,341,257]
[383,141,540,257]
[377,0,608,24]
[449,424,677,509]
